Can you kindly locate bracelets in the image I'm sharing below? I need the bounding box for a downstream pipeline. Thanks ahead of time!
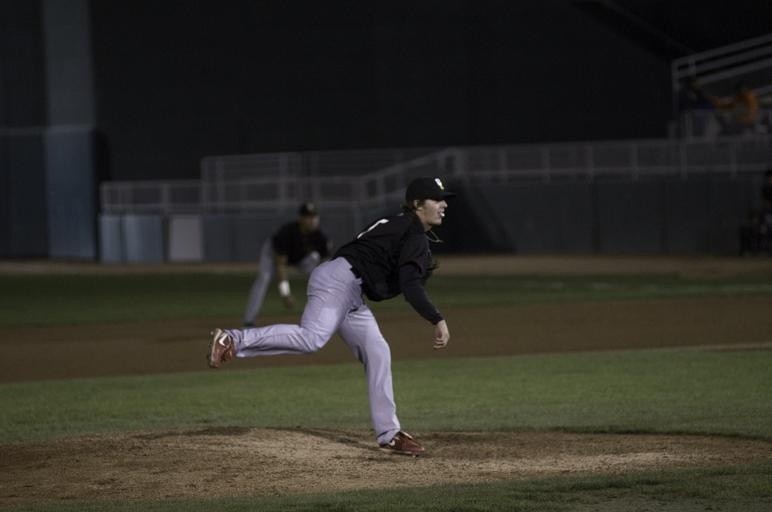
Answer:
[277,279,291,298]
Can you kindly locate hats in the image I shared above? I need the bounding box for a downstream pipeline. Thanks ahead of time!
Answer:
[297,203,318,217]
[405,177,453,200]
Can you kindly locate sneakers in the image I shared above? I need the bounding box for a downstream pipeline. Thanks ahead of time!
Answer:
[207,327,234,368]
[380,430,425,456]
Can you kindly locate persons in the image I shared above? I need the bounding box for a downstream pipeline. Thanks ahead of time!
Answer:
[674,78,722,136]
[243,201,333,330]
[758,167,770,204]
[691,79,760,134]
[205,177,456,457]
[749,193,772,255]
